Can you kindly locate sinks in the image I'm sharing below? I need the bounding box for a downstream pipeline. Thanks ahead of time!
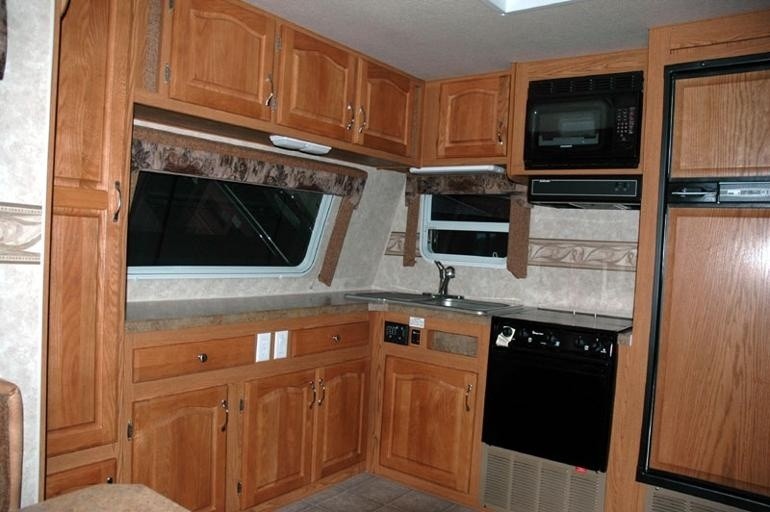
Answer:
[433,296,524,313]
[342,289,432,303]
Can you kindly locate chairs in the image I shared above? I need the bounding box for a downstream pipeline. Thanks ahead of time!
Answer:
[0,379,195,512]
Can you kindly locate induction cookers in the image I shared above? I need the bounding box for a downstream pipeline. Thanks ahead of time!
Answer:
[489,308,632,332]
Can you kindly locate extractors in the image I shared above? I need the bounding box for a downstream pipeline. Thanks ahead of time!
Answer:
[525,176,641,211]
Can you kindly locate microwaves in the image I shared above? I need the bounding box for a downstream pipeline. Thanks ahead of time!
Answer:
[524,70,644,169]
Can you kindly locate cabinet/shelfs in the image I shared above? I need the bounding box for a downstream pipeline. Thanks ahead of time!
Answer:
[277,16,424,166]
[45,0,133,455]
[134,382,240,512]
[423,69,511,166]
[125,310,373,381]
[46,456,133,503]
[240,354,373,510]
[168,1,276,124]
[373,355,485,496]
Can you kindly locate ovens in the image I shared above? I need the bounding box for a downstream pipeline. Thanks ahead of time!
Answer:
[482,342,618,473]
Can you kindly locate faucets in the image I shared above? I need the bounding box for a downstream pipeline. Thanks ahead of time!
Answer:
[431,256,457,296]
